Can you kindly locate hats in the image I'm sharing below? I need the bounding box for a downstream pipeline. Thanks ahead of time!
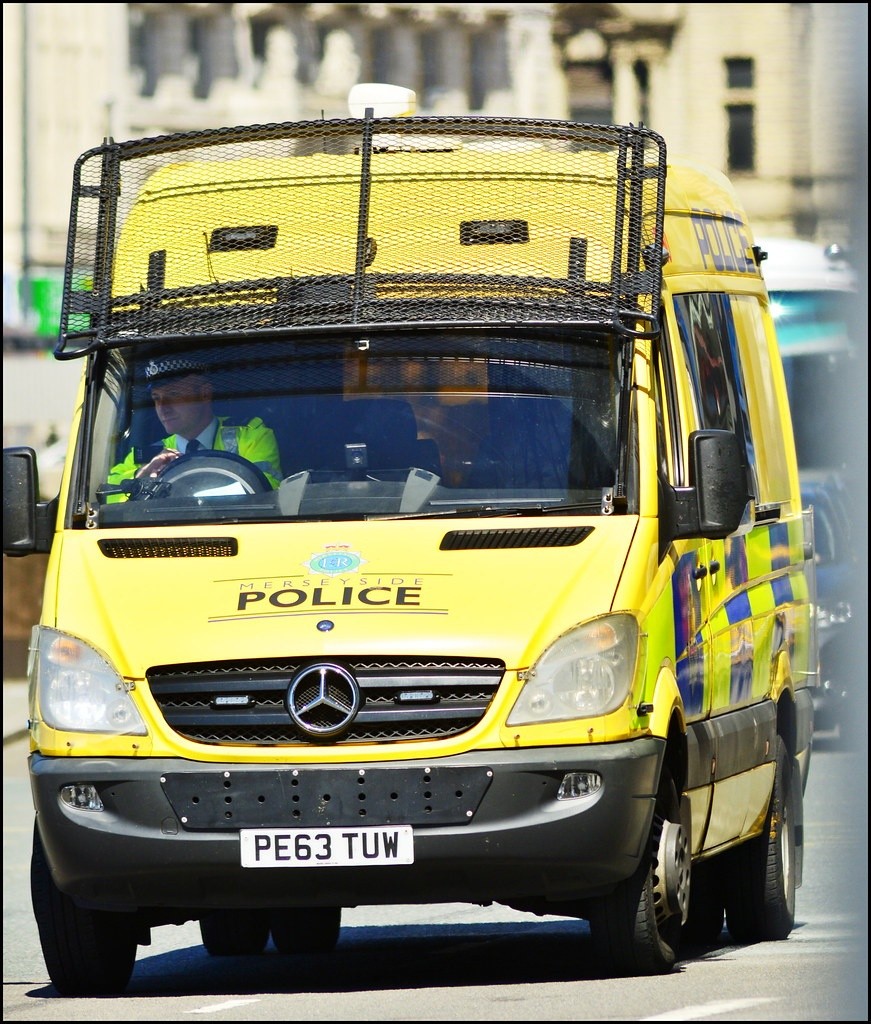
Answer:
[145,348,213,391]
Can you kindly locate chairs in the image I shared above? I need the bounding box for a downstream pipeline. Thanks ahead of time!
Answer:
[317,397,445,489]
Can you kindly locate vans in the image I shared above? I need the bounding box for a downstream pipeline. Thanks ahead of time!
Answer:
[3,81,820,993]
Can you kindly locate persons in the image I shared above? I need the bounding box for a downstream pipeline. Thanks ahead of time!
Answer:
[107,352,282,504]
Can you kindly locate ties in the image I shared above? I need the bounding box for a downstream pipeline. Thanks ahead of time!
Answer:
[185,439,199,454]
